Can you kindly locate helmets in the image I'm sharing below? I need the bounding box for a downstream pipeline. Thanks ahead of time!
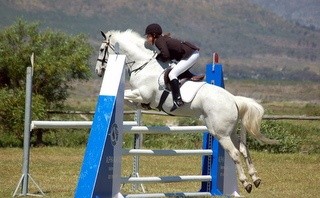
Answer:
[146,24,162,35]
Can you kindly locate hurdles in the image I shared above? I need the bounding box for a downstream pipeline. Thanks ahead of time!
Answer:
[12,66,146,197]
[73,54,238,198]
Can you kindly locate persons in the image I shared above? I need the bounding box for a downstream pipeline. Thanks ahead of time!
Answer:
[145,23,200,111]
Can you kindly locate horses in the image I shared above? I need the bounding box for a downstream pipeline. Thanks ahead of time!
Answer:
[94,28,281,194]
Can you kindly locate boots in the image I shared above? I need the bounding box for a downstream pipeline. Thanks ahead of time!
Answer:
[170,79,184,112]
